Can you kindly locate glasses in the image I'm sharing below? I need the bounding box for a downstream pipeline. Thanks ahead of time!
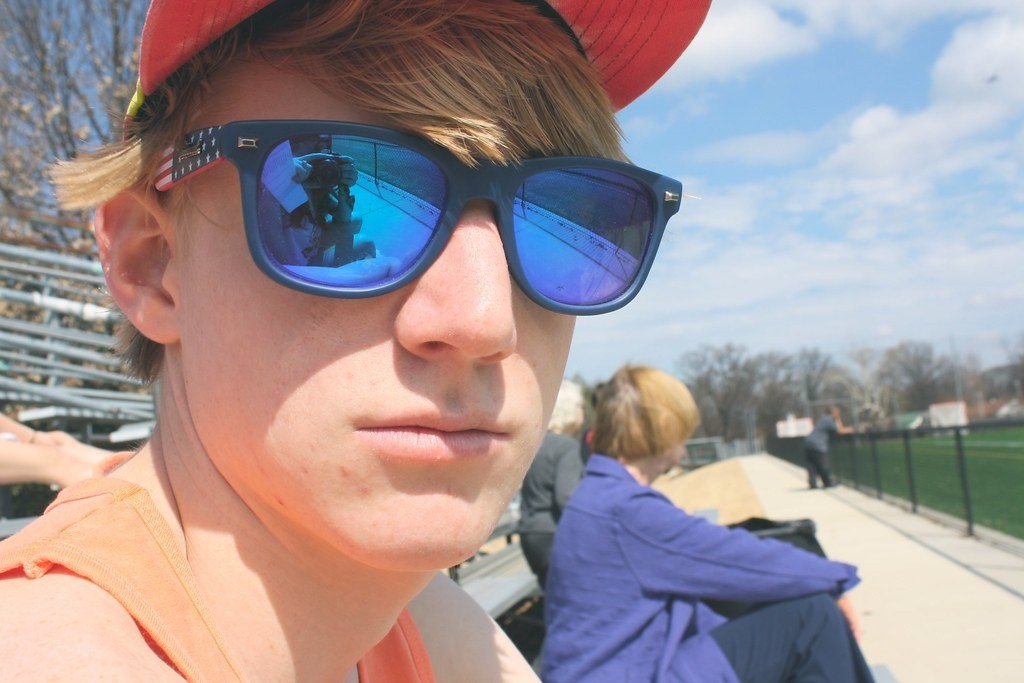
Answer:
[149,117,684,316]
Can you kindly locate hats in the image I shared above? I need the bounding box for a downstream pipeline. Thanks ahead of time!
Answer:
[121,0,713,140]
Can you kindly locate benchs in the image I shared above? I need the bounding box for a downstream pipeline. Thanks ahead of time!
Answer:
[443,495,545,683]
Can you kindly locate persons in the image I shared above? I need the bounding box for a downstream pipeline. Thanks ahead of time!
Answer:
[540,367,875,683]
[286,135,377,267]
[516,377,587,587]
[0,0,714,683]
[805,405,854,489]
